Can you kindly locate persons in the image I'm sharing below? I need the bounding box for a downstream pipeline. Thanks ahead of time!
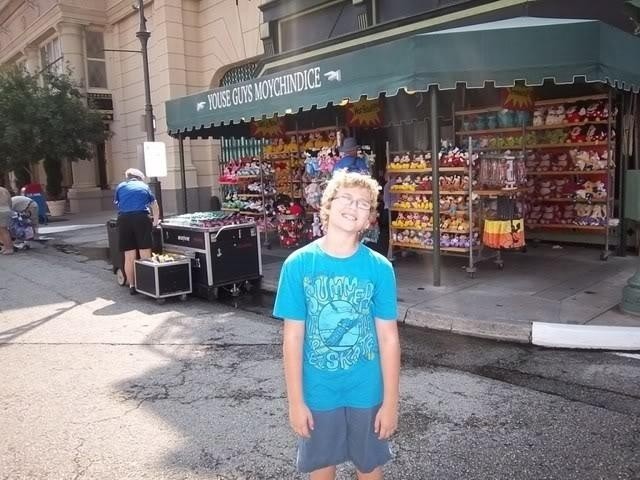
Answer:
[112,168,160,295]
[11,196,40,241]
[0,177,14,255]
[272,167,404,479]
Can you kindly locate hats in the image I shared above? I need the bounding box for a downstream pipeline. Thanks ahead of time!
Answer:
[337,137,362,152]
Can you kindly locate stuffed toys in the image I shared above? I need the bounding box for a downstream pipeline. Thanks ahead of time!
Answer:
[221,103,619,251]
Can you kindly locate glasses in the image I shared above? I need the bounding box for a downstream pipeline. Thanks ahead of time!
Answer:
[330,193,371,212]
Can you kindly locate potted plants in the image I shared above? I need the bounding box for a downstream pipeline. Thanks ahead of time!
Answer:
[46,192,67,217]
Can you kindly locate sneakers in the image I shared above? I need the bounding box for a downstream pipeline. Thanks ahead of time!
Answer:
[130,286,138,295]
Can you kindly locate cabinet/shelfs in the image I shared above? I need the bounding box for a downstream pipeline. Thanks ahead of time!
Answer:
[524,89,616,260]
[450,100,523,253]
[386,136,505,278]
[218,149,295,250]
[296,146,380,249]
[276,126,350,150]
[218,216,264,278]
